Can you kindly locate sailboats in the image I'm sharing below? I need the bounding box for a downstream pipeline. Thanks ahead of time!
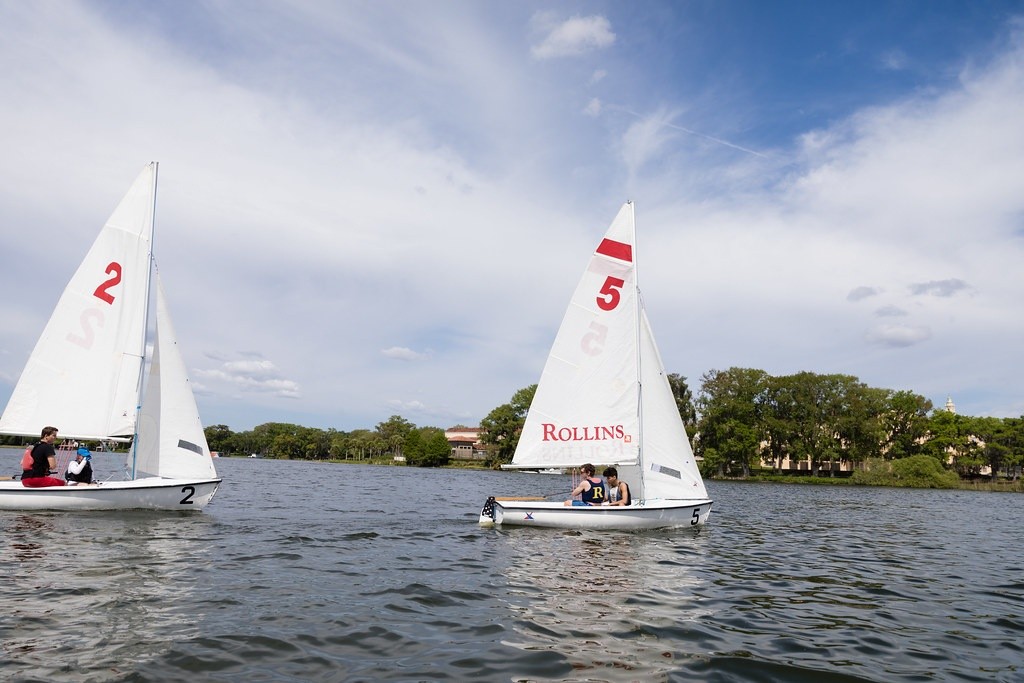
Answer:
[477,198,717,533]
[0,159,224,513]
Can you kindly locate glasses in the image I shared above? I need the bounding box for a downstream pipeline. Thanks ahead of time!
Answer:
[581,471,588,474]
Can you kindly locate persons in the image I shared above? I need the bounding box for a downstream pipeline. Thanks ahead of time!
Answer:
[64,447,98,486]
[602,467,631,506]
[21,426,68,487]
[564,464,608,506]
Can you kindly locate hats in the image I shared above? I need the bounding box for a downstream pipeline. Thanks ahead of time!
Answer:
[77,448,92,457]
